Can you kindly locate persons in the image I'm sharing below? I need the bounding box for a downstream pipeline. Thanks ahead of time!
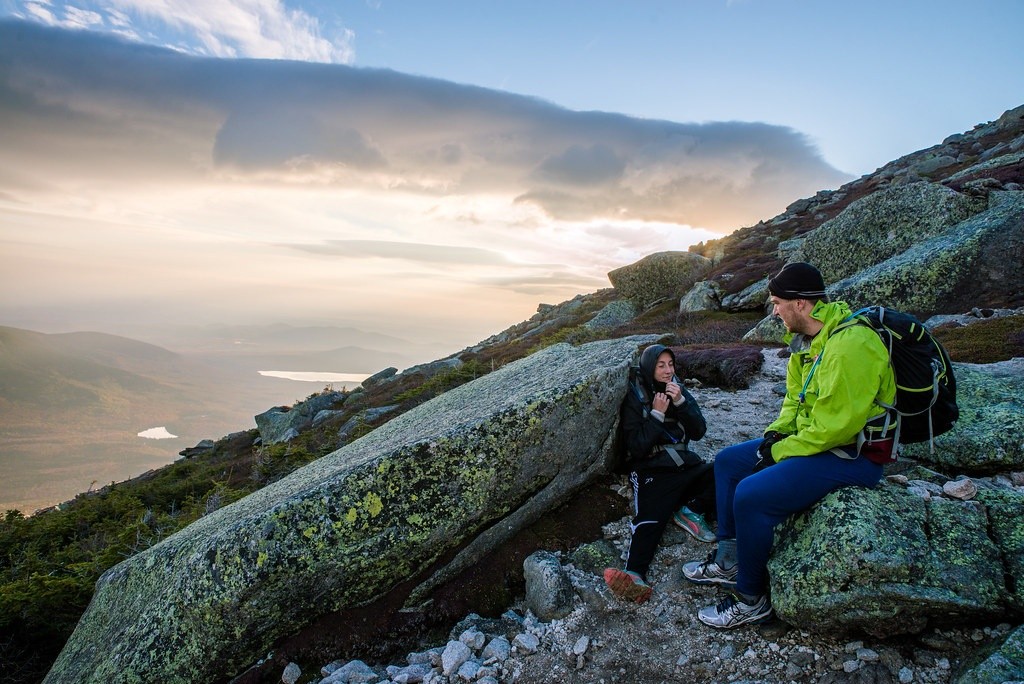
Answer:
[683,263,896,628]
[604,345,715,604]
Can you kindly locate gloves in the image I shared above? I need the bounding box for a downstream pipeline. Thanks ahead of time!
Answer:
[754,432,784,468]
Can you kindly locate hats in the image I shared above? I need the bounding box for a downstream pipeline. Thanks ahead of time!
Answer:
[769,263,827,299]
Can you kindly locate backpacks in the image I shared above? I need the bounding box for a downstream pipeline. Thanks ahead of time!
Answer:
[833,306,960,459]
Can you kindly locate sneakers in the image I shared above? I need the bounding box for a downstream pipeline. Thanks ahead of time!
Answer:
[674,506,716,543]
[604,567,651,602]
[681,549,737,589]
[697,588,772,629]
